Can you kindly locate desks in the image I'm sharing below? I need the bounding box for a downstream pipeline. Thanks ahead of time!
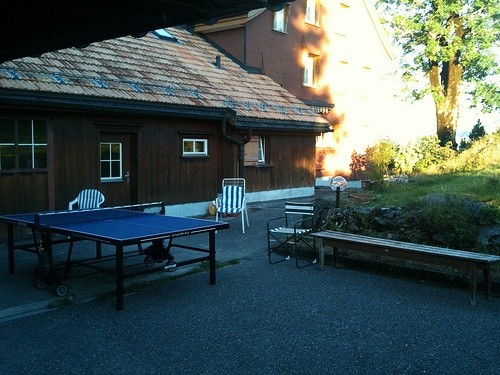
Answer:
[0,208,230,312]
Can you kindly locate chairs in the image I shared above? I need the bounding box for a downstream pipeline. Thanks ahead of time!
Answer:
[266,201,317,269]
[212,178,249,234]
[68,187,105,211]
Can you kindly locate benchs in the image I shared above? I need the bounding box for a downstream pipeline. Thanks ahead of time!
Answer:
[309,229,500,306]
